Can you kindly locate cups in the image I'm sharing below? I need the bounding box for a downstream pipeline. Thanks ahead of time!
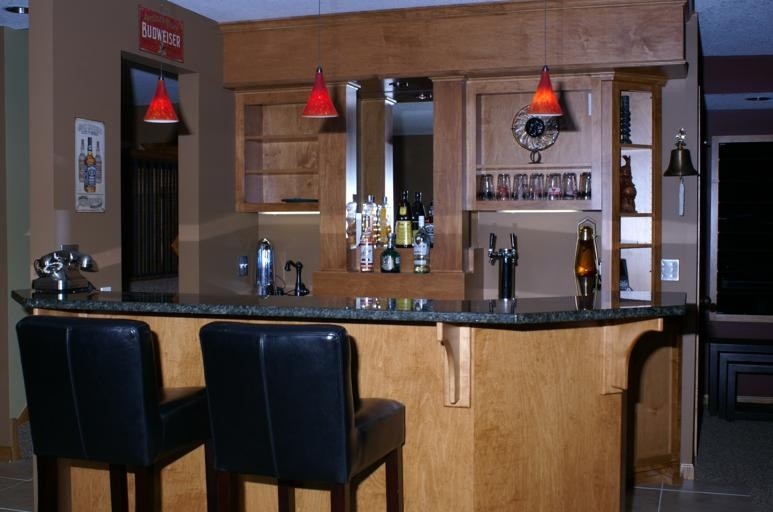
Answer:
[478,172,591,199]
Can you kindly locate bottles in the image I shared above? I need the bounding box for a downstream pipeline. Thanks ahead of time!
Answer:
[619,259,628,291]
[78,137,102,192]
[344,191,434,273]
[620,95,631,143]
[359,297,431,314]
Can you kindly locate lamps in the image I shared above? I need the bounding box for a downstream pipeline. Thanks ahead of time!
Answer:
[524,0,567,118]
[298,0,341,123]
[661,125,698,215]
[137,7,186,128]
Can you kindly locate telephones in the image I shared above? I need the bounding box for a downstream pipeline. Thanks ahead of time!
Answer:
[31,249,97,291]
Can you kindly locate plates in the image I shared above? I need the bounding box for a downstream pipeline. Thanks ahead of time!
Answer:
[280,197,319,204]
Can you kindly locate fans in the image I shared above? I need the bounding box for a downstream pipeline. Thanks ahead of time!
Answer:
[509,103,559,163]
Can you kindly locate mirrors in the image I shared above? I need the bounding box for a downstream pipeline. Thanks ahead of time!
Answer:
[355,87,436,250]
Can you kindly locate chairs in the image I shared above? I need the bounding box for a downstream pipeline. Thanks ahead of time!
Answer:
[194,317,408,510]
[12,312,215,511]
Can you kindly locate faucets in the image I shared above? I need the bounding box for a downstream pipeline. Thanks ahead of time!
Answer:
[284,256,309,296]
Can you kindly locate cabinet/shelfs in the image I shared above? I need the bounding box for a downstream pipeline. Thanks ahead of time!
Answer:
[232,87,318,212]
[464,74,602,214]
[601,71,669,310]
